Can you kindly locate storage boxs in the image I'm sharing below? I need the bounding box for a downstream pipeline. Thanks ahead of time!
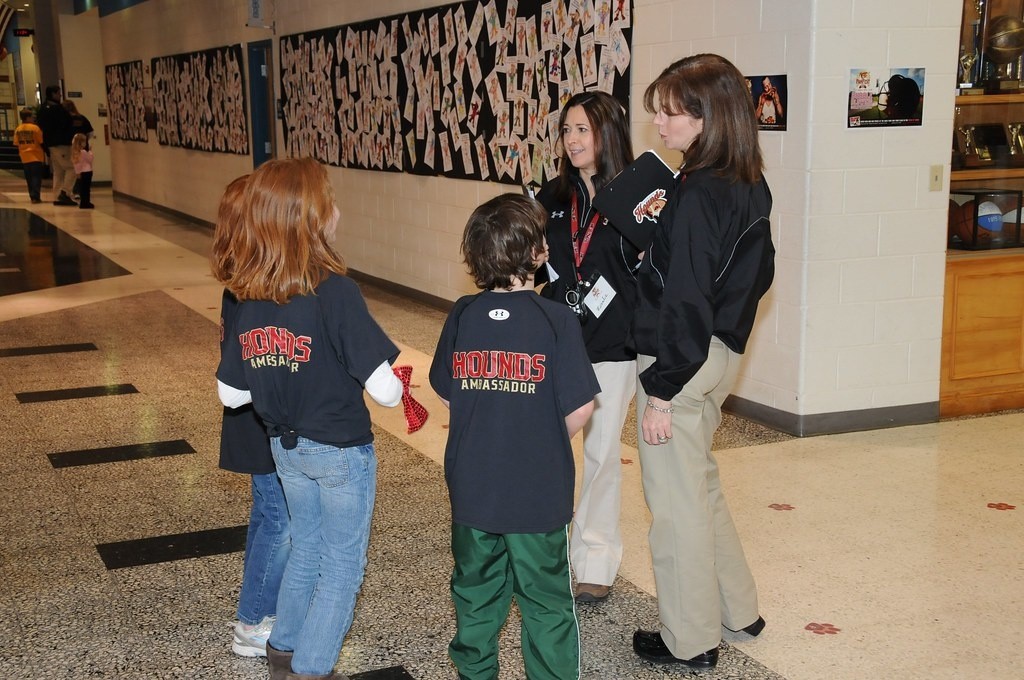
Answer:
[948,188,1024,250]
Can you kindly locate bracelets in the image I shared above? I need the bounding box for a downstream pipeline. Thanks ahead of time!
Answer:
[647,400,674,413]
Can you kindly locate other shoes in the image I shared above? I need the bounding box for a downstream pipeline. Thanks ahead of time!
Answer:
[79,202,94,208]
[31,198,41,203]
[75,194,80,198]
[53,190,78,206]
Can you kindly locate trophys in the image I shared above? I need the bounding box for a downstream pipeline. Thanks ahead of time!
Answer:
[952,0,1024,169]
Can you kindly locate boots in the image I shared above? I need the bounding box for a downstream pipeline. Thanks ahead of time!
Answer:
[267,639,350,680]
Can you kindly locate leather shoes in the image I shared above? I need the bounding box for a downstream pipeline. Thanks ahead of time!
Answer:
[633,629,719,669]
[742,615,766,636]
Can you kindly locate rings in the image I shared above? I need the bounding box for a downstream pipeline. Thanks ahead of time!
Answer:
[658,437,667,442]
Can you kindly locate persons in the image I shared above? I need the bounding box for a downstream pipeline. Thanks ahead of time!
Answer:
[534,91,642,602]
[210,173,292,657]
[429,193,601,680]
[630,54,775,668]
[13,110,43,204]
[71,134,95,209]
[755,77,783,124]
[36,85,94,206]
[215,157,403,680]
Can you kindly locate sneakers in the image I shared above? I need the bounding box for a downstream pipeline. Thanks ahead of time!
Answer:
[232,616,277,657]
[575,583,610,601]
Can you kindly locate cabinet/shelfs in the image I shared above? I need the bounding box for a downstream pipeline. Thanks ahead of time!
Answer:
[939,0,1024,417]
